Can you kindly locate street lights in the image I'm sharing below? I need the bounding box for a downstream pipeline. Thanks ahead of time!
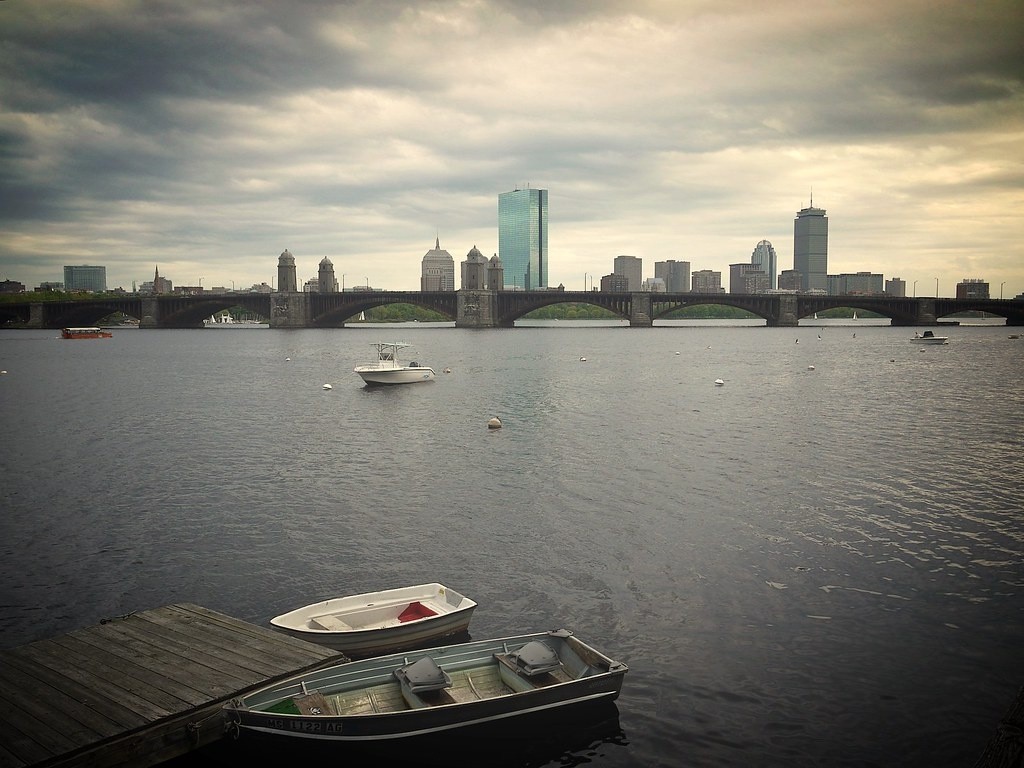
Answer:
[935,278,938,299]
[1001,282,1006,301]
[914,281,918,297]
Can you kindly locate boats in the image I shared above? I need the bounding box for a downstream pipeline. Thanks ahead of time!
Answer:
[221,629,629,744]
[354,342,436,384]
[62,327,113,339]
[268,582,477,660]
[910,331,949,345]
